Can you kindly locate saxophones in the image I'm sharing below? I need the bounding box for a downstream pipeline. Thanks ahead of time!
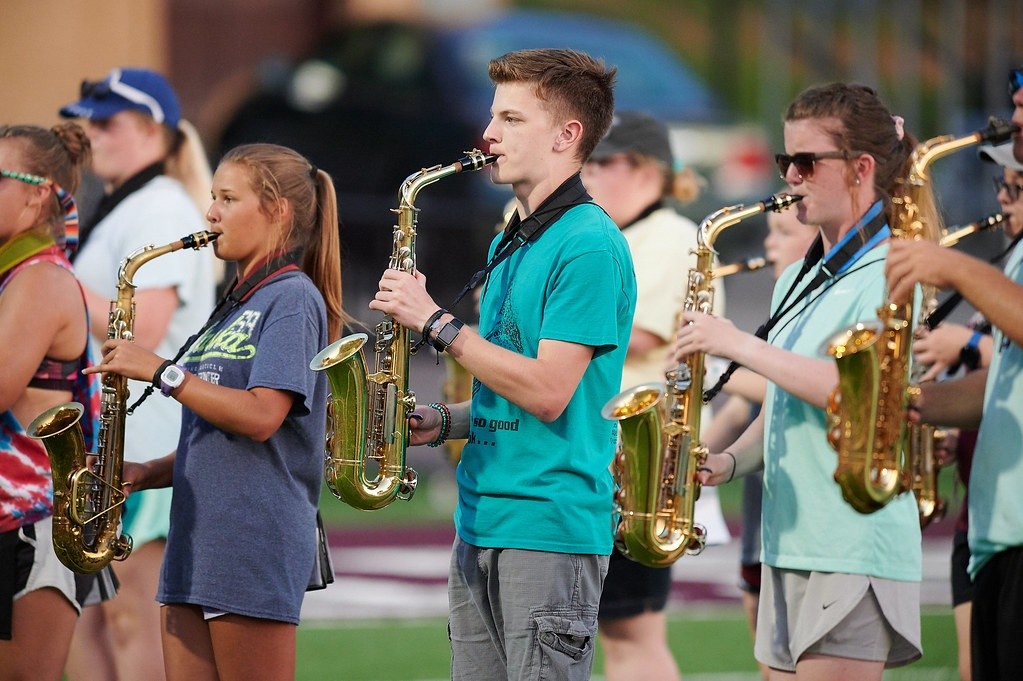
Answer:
[306,146,503,515]
[903,211,1011,528]
[601,191,807,568]
[429,201,518,465]
[824,113,1018,520]
[22,227,221,576]
[715,257,772,279]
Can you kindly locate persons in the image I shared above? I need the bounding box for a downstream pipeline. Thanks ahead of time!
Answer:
[697,67,1023,681]
[561,116,728,680]
[1,67,344,681]
[370,48,638,680]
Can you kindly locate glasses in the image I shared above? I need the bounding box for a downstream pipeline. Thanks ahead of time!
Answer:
[1008,68,1023,96]
[80,81,122,101]
[774,149,886,180]
[586,156,633,167]
[992,175,1023,200]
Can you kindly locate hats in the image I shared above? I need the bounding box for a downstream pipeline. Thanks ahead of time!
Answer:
[977,142,1023,171]
[585,113,672,159]
[57,68,182,131]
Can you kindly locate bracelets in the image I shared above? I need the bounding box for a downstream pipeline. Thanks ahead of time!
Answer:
[721,450,736,484]
[421,308,449,344]
[152,360,177,390]
[427,401,451,448]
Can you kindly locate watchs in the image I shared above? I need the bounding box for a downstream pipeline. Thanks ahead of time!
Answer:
[946,332,983,378]
[160,363,189,398]
[430,319,464,353]
